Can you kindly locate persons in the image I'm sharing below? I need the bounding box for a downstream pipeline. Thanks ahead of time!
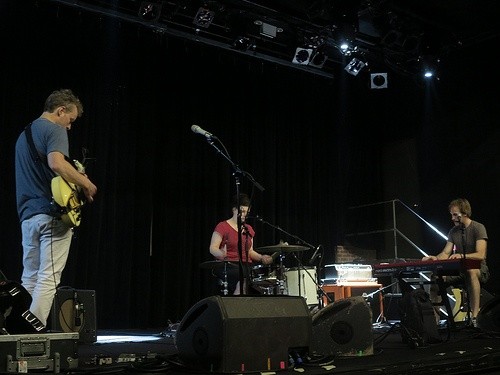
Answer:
[422,197,489,325]
[15,87,97,327]
[209,192,272,277]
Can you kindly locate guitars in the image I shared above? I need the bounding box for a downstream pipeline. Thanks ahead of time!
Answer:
[51,147,88,227]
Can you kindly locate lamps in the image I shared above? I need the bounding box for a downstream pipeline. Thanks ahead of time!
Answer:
[368,65,388,89]
[291,35,314,65]
[308,51,329,70]
[192,3,215,29]
[343,49,366,77]
[137,0,165,23]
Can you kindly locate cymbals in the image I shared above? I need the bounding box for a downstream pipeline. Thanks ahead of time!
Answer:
[200,261,227,269]
[256,245,310,253]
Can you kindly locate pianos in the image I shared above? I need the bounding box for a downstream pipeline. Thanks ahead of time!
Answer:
[372,258,481,347]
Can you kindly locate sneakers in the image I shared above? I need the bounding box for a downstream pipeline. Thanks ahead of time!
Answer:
[470,317,477,327]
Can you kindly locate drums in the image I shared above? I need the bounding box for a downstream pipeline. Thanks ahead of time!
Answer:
[280,266,320,309]
[251,265,285,289]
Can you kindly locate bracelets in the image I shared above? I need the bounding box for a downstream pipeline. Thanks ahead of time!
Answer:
[460,253,463,259]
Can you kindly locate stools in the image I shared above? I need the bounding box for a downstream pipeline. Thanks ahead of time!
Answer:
[453,283,474,329]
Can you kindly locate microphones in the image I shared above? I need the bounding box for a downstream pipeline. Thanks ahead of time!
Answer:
[458,214,462,229]
[191,125,218,139]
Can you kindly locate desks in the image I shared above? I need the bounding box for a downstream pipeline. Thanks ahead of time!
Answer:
[320,282,384,322]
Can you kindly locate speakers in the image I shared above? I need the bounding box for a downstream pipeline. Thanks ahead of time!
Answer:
[285,266,318,304]
[7,286,97,343]
[309,296,373,357]
[172,294,312,375]
